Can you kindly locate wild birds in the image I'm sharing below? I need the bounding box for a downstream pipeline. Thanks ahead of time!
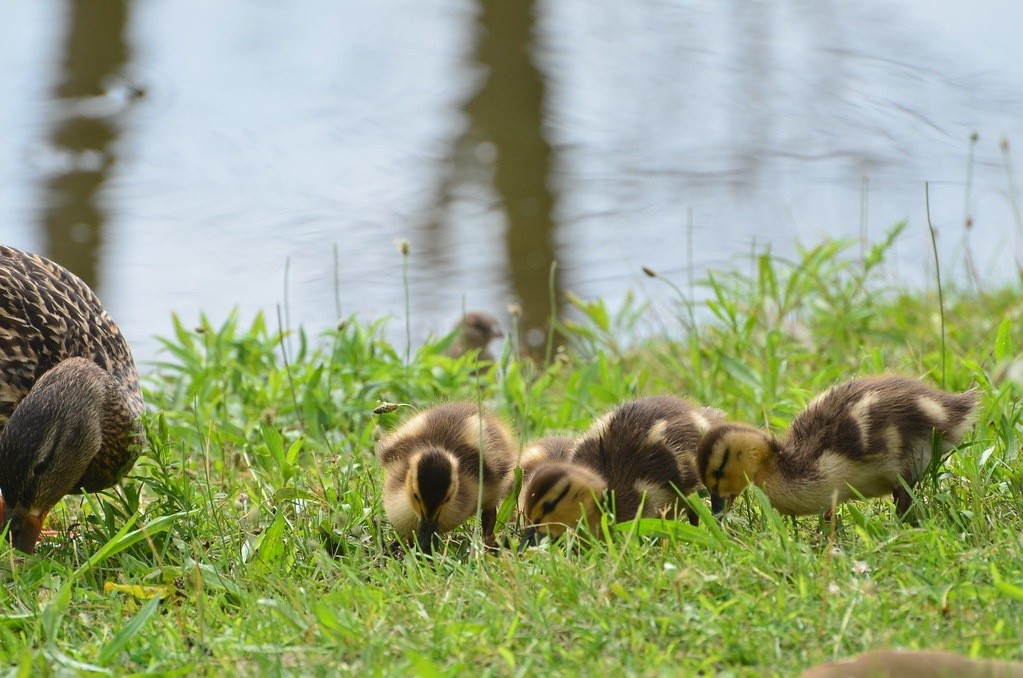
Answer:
[0,242,146,556]
[435,309,504,377]
[376,394,522,557]
[499,390,728,557]
[694,366,985,539]
